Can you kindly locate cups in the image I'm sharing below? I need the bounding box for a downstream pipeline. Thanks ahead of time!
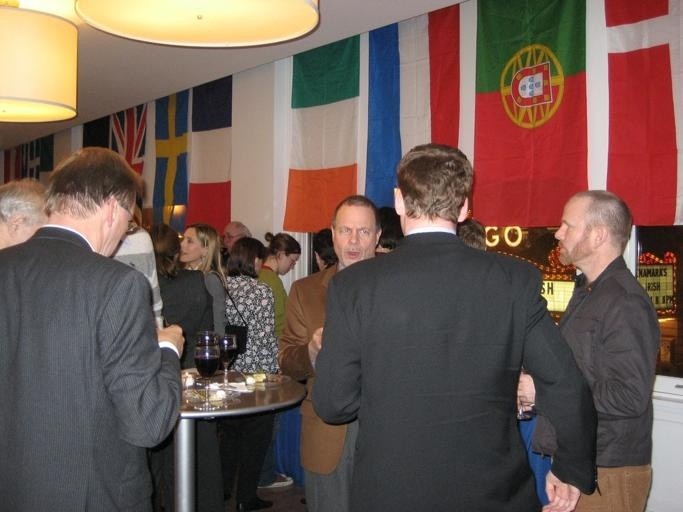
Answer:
[193,331,222,410]
[218,334,237,390]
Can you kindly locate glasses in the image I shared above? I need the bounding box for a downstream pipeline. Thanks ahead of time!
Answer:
[118,198,144,234]
[288,256,297,267]
[220,233,243,241]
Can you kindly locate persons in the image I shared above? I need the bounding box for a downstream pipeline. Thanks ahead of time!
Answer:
[276,193,387,511]
[376,206,403,255]
[309,142,604,512]
[515,188,664,511]
[223,236,282,511]
[257,232,303,349]
[219,220,253,264]
[456,216,487,253]
[179,222,227,345]
[146,222,214,367]
[0,178,48,250]
[310,228,338,272]
[0,147,186,511]
[111,219,164,338]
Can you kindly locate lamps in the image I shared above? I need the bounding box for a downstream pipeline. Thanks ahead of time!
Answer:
[74,0,322,48]
[0,0,80,123]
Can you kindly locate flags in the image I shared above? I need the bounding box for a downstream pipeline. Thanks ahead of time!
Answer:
[22,139,40,179]
[463,0,592,230]
[363,2,464,213]
[5,145,21,184]
[81,113,113,150]
[282,30,360,237]
[110,102,150,176]
[186,74,233,237]
[36,133,54,171]
[601,0,682,228]
[151,87,191,237]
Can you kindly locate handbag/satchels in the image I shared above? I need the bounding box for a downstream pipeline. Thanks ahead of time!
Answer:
[209,271,248,354]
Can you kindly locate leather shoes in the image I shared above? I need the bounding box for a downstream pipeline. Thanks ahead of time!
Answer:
[258,473,293,489]
[236,498,275,511]
